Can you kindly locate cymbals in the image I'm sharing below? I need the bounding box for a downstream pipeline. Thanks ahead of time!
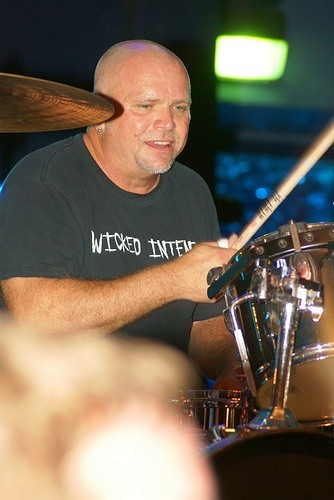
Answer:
[1,73,116,135]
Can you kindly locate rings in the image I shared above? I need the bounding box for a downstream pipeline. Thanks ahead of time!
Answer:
[216,237,229,250]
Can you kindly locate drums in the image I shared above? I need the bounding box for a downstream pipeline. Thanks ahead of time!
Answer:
[200,425,333,499]
[220,221,334,426]
[163,386,250,434]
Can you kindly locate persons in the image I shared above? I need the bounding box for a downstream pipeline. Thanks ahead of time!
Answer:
[0,38,241,382]
